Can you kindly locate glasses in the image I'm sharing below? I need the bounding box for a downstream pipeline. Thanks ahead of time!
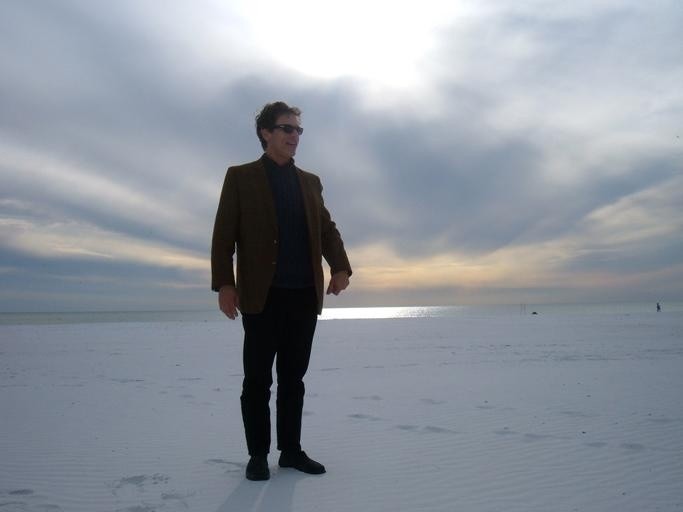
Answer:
[273,124,304,135]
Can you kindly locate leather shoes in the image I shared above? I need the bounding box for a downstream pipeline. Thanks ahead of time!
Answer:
[246,458,270,480]
[279,451,326,474]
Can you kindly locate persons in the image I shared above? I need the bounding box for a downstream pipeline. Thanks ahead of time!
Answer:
[212,101,353,482]
